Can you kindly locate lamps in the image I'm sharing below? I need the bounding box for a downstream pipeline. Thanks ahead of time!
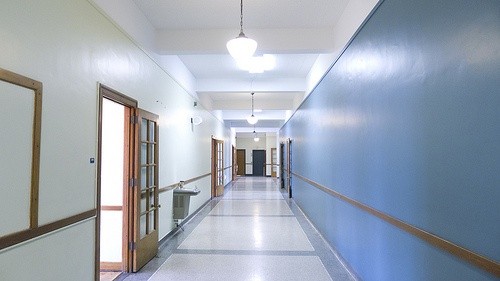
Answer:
[226,0,258,71]
[190,115,203,125]
[252,124,259,141]
[248,91,258,124]
